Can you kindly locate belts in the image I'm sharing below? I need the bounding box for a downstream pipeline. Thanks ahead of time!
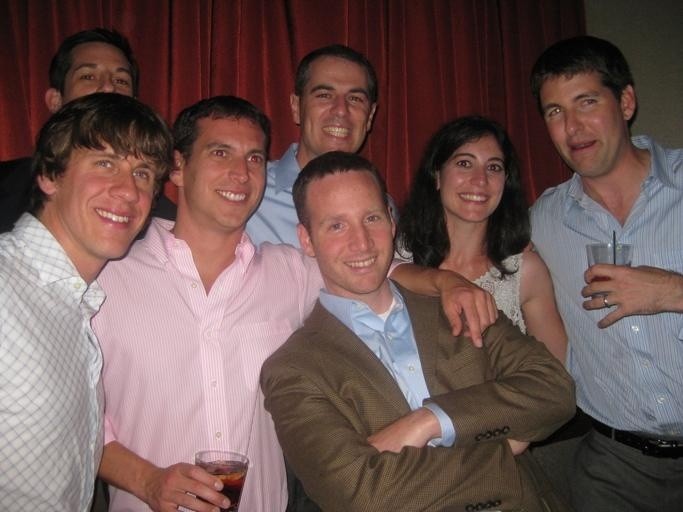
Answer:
[593,420,683,459]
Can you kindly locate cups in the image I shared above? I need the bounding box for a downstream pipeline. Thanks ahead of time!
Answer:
[582,241,635,300]
[193,449,249,512]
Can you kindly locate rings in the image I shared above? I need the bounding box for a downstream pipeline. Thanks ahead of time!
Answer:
[602,294,610,308]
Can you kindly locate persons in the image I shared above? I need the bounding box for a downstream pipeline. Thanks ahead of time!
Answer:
[391,113,568,370]
[525,34,682,512]
[0,26,176,242]
[258,150,577,511]
[243,43,400,511]
[0,92,176,512]
[94,95,500,511]
